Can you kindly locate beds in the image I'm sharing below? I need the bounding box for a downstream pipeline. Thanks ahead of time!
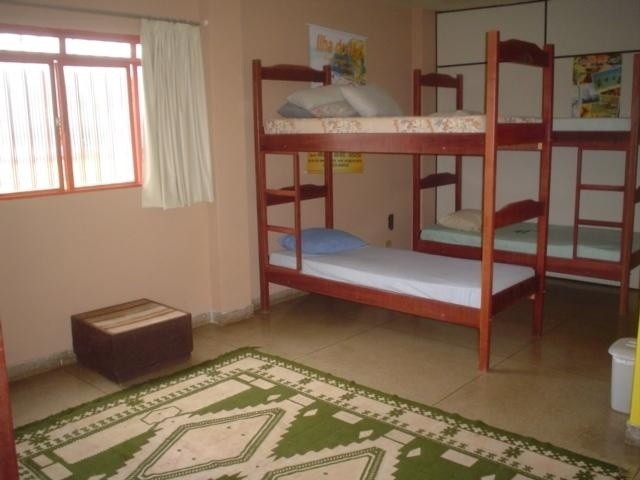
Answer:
[412,52,640,318]
[252,32,556,369]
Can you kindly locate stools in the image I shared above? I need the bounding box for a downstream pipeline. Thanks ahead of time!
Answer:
[71,297,193,384]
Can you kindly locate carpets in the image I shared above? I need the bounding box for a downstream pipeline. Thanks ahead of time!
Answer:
[14,347,628,480]
